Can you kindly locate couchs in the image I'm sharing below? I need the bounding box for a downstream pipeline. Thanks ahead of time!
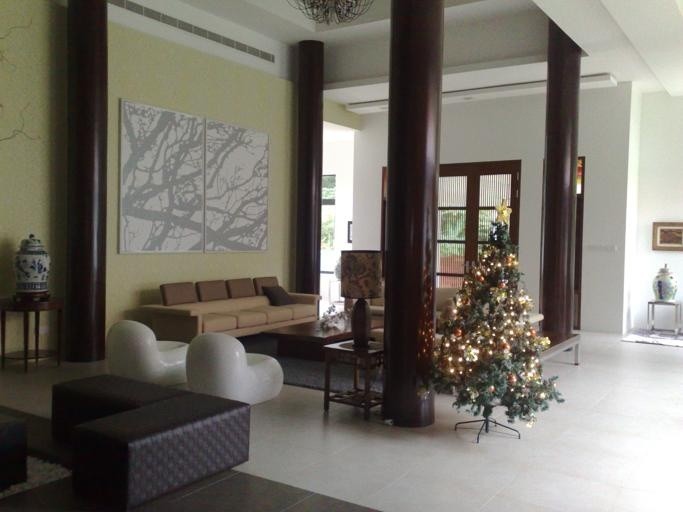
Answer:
[343,285,459,333]
[136,276,322,344]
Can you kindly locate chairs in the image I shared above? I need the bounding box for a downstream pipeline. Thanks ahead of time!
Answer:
[103,320,284,405]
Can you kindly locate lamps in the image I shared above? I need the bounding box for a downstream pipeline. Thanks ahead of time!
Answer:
[286,0,373,26]
[340,250,382,351]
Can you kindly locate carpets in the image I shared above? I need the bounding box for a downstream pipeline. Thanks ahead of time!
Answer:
[238,335,384,394]
[620,328,683,347]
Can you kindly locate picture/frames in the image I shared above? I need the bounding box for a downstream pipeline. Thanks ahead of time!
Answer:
[652,222,683,251]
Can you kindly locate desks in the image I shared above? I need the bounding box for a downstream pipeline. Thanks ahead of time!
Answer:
[647,300,681,339]
[0,297,64,373]
[321,339,383,420]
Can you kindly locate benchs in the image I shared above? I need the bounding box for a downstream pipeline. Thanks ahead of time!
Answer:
[49,373,249,511]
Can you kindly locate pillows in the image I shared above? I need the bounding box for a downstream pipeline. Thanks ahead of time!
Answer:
[262,285,295,306]
